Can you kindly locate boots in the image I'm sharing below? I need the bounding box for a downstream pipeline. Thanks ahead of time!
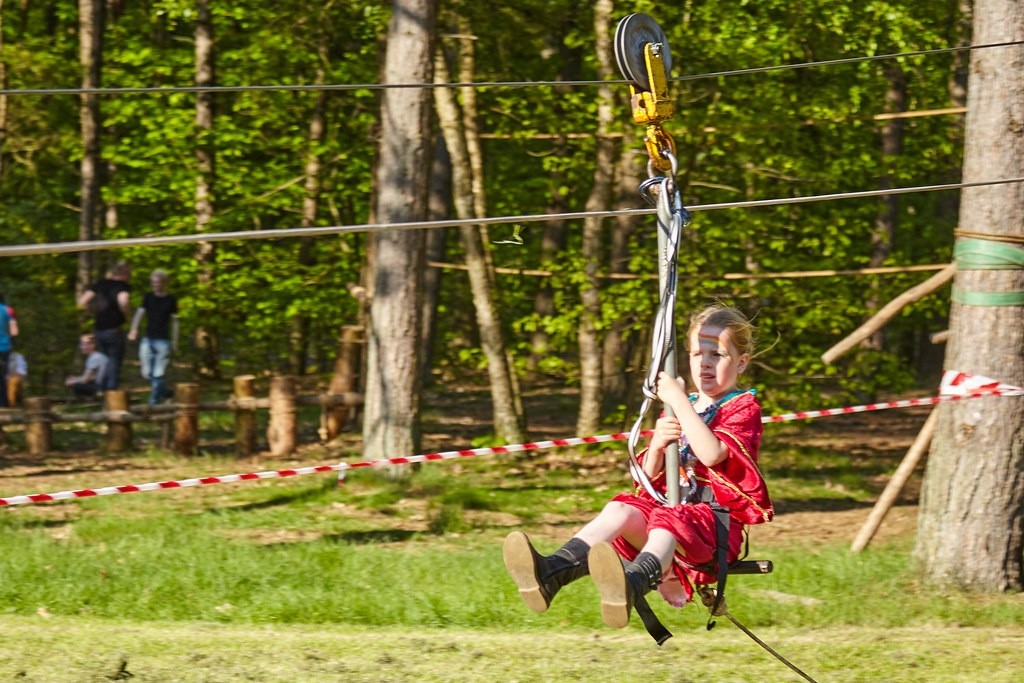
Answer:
[149,375,174,404]
[502,531,590,612]
[587,540,662,628]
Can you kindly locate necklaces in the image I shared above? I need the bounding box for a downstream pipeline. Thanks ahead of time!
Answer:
[680,390,745,464]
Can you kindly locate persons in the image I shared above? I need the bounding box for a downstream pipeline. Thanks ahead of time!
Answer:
[127,270,178,405]
[0,291,27,409]
[66,335,109,401]
[79,266,130,398]
[503,307,774,646]
